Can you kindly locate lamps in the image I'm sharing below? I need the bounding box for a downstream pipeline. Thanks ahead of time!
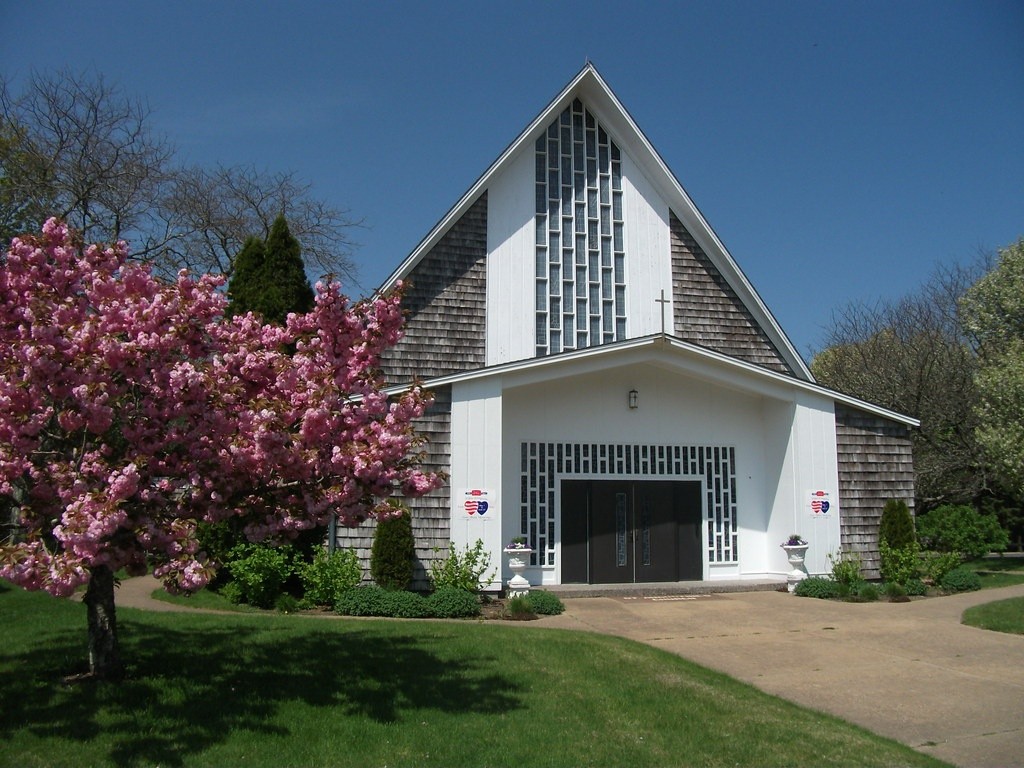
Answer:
[629,389,639,409]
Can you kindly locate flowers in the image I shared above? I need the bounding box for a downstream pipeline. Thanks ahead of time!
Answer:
[780,534,808,546]
[505,536,530,549]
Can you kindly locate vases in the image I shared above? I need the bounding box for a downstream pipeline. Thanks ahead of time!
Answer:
[783,545,810,594]
[503,548,533,598]
